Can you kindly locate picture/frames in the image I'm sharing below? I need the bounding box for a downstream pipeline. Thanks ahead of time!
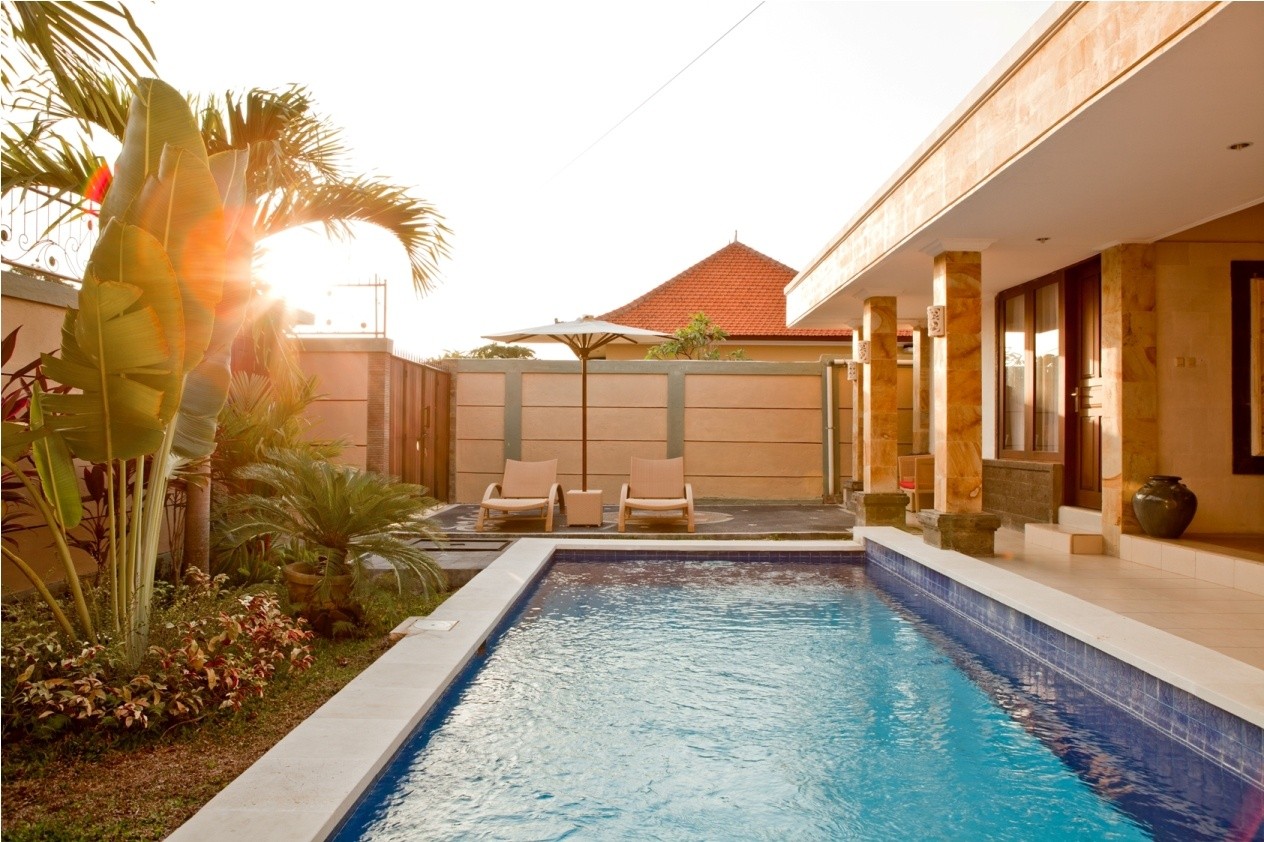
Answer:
[1230,259,1264,475]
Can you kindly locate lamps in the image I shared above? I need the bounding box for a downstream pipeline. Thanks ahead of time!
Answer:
[848,361,858,381]
[928,305,944,337]
[855,341,870,364]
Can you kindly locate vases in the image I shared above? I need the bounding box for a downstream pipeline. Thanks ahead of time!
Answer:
[1132,475,1199,538]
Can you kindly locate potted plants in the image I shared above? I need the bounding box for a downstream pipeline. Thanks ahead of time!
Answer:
[227,439,453,620]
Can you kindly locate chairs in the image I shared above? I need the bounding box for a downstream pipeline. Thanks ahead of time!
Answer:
[476,459,565,532]
[897,454,934,512]
[618,456,695,533]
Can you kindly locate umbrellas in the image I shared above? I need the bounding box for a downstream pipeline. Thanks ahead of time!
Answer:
[482,316,679,492]
